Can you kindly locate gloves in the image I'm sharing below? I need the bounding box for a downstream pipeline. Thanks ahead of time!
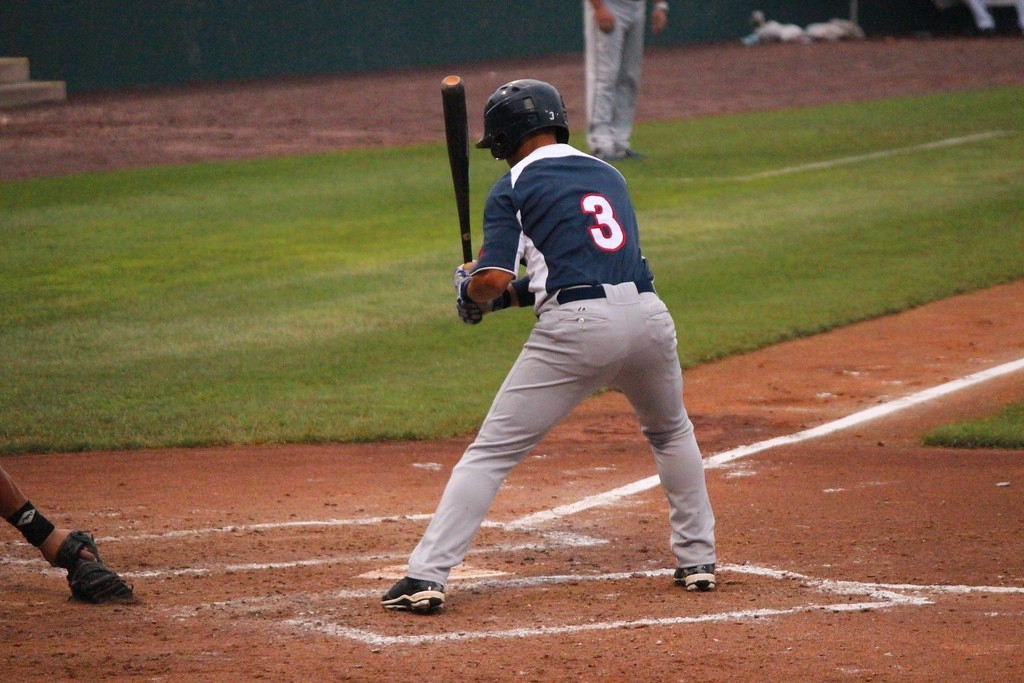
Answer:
[453,259,477,304]
[456,288,512,325]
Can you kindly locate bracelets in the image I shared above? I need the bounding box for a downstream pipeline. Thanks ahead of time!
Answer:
[654,2,670,11]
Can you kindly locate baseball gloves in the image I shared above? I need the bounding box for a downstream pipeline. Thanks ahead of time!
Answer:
[63,523,133,604]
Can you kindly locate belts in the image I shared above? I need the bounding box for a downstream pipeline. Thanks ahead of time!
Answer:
[535,279,656,320]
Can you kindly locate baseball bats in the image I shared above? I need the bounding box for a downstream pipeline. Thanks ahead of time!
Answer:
[441,75,480,310]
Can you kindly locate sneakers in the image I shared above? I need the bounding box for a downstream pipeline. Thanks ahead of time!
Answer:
[380,577,445,614]
[673,563,716,591]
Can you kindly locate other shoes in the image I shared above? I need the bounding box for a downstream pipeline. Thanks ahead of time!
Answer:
[739,33,761,47]
[614,142,642,160]
[587,147,628,161]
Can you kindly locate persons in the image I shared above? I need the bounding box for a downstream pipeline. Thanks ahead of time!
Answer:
[380,75,722,615]
[578,0,668,164]
[0,466,97,569]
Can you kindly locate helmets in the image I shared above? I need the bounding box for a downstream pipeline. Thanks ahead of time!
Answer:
[475,78,569,160]
